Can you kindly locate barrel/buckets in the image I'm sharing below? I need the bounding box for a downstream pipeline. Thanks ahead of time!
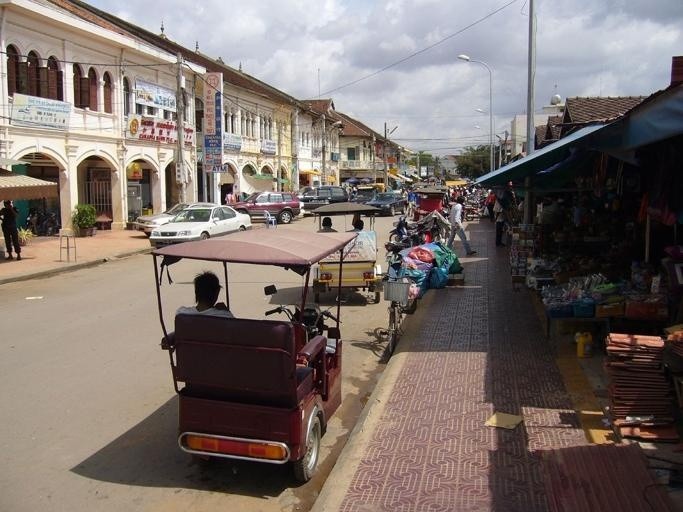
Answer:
[576,331,593,359]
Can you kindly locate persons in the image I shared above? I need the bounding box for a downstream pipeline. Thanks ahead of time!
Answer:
[443,195,476,256]
[344,220,364,232]
[238,191,248,201]
[400,176,582,248]
[313,217,337,233]
[175,272,236,318]
[0,198,22,261]
[223,190,234,205]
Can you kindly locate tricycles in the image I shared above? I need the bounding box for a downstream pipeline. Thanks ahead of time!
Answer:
[311,200,384,305]
[149,225,361,486]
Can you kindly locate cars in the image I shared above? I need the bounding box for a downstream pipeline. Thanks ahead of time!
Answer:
[297,184,377,209]
[149,205,253,249]
[135,202,218,238]
[366,192,408,217]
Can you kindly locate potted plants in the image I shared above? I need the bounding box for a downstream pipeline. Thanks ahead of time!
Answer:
[75,204,98,237]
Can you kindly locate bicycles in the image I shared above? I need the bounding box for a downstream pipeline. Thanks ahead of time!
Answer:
[375,273,421,355]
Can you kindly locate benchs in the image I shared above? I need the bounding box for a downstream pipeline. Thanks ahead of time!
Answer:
[163,314,327,402]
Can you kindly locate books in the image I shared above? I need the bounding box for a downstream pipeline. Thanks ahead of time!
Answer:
[581,272,610,290]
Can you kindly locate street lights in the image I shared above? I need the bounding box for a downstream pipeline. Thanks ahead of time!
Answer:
[456,54,496,219]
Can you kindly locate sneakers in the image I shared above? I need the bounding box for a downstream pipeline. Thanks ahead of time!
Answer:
[467,251,477,255]
[17,254,21,260]
[8,254,13,259]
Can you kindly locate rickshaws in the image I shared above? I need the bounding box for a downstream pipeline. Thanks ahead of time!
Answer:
[411,181,452,224]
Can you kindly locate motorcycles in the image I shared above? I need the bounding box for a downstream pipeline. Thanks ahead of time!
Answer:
[389,215,432,247]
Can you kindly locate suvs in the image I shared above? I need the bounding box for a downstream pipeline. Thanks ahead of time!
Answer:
[226,192,301,224]
